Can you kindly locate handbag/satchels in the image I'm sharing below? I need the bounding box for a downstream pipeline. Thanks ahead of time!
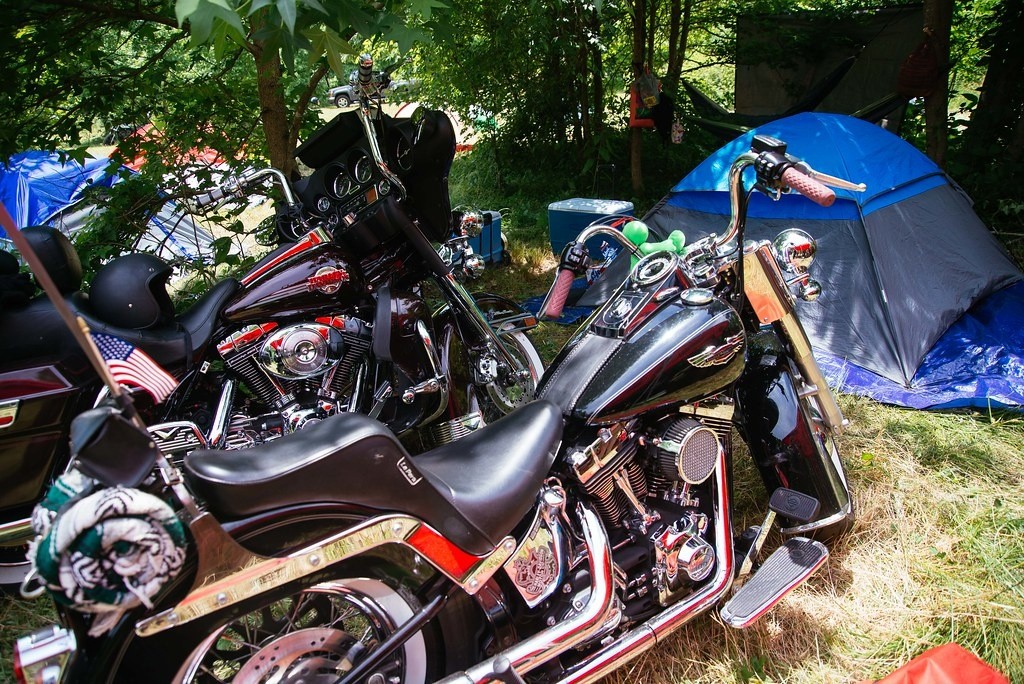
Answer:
[629,63,661,128]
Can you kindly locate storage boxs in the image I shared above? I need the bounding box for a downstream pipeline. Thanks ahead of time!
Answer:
[547,198,634,260]
[452,210,503,264]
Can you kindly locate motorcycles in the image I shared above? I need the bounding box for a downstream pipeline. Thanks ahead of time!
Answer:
[20,134,868,684]
[0,57,546,589]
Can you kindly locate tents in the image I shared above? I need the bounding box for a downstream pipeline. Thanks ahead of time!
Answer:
[387,95,499,152]
[640,112,1024,390]
[0,122,273,285]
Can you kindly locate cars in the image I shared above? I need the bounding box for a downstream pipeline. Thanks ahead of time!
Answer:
[328,79,423,108]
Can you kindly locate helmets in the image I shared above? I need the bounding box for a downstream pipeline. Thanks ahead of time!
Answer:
[18,226,82,300]
[89,254,174,329]
